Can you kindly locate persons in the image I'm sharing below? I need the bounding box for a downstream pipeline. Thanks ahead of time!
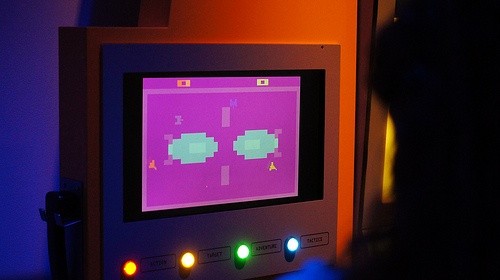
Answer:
[330,0,499,280]
